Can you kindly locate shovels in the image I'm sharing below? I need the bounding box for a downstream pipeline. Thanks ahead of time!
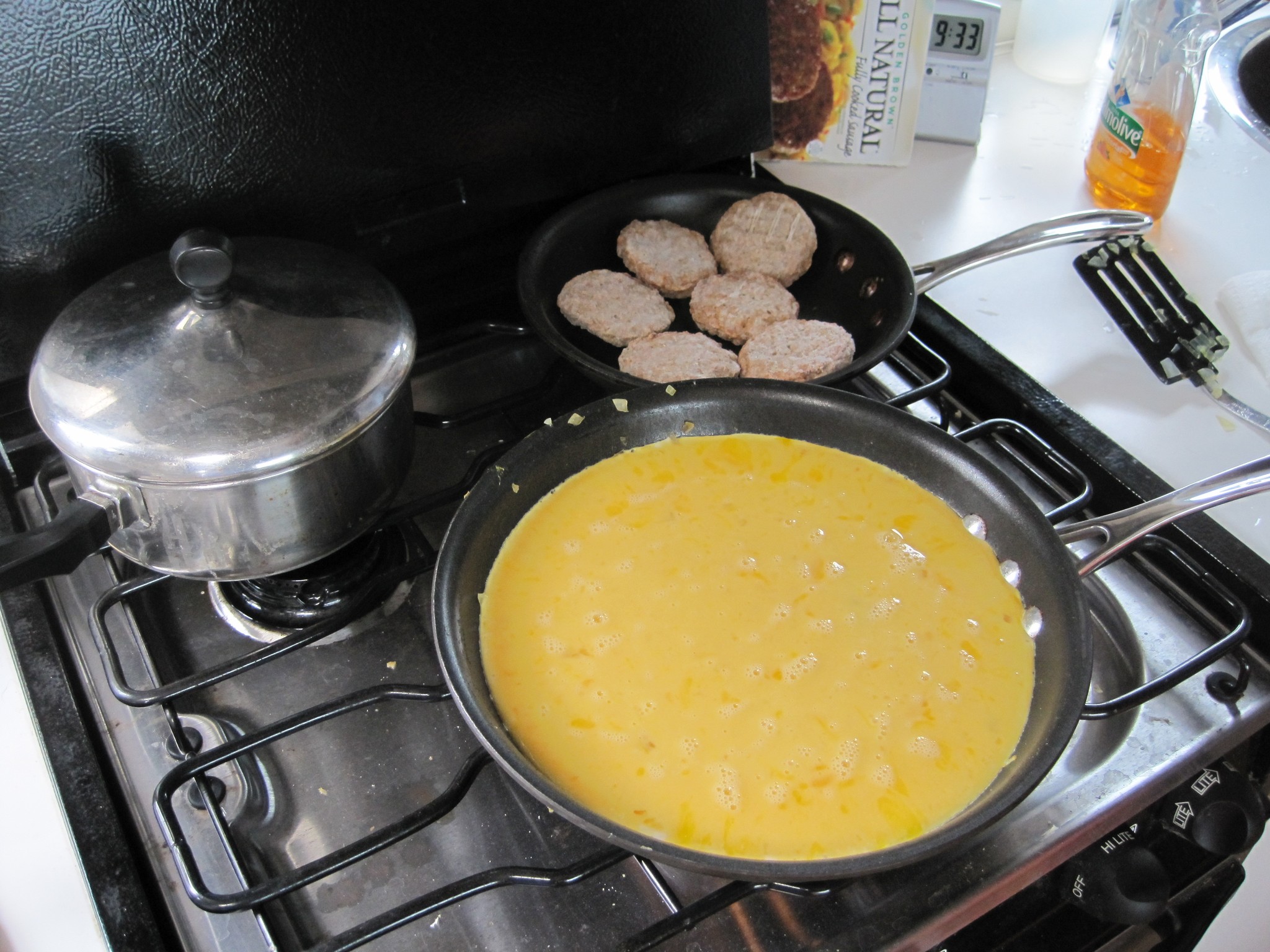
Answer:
[1072,234,1270,433]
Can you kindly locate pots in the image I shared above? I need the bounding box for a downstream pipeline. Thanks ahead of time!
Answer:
[0,229,417,583]
[429,375,1270,885]
[515,174,1151,398]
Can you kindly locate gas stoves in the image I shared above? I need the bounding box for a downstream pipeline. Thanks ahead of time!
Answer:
[0,290,1270,952]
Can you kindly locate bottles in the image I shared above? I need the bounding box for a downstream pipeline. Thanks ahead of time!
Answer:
[1087,0,1221,222]
